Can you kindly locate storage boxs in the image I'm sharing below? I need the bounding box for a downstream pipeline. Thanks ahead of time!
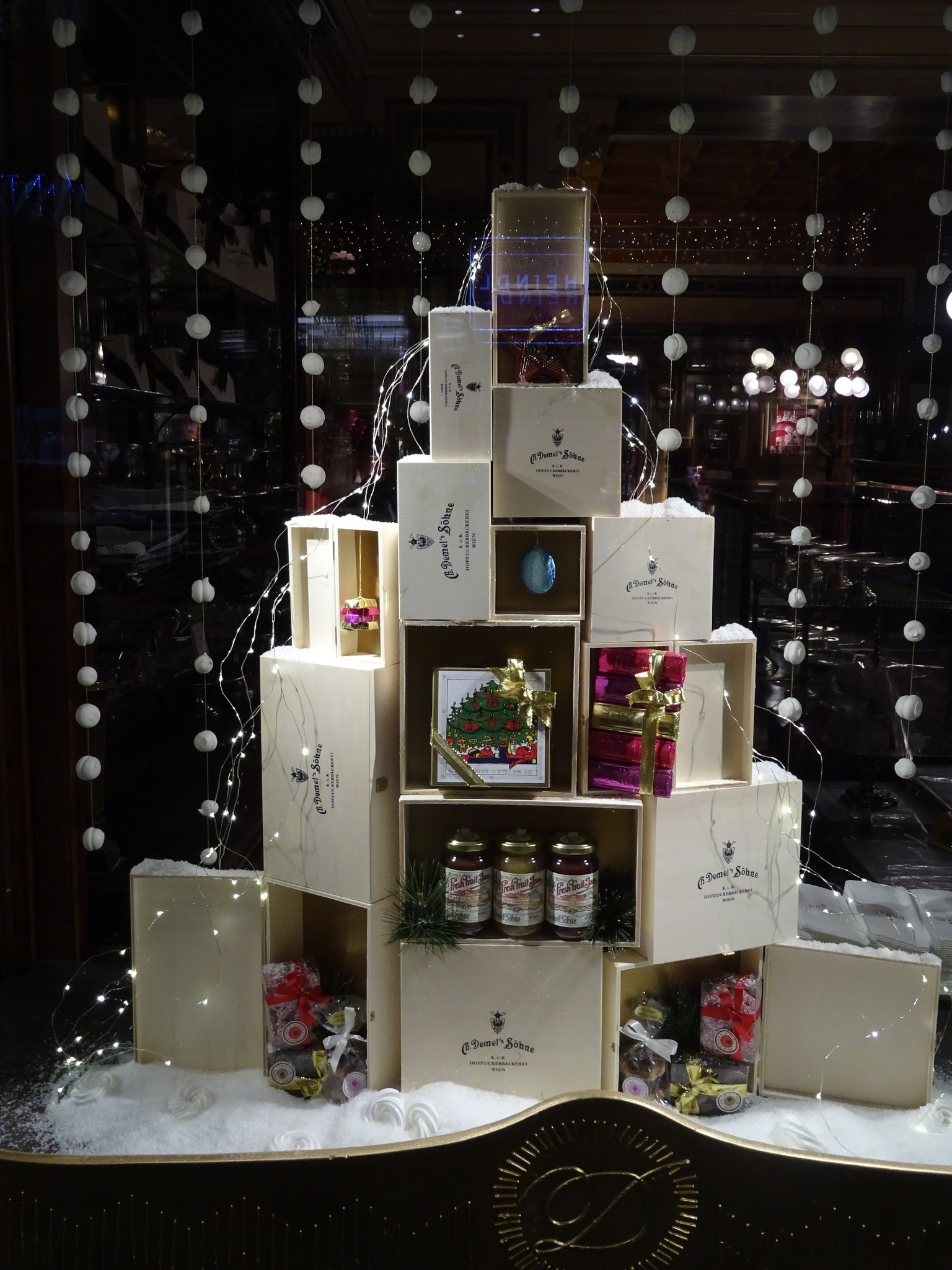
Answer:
[128,184,943,1116]
